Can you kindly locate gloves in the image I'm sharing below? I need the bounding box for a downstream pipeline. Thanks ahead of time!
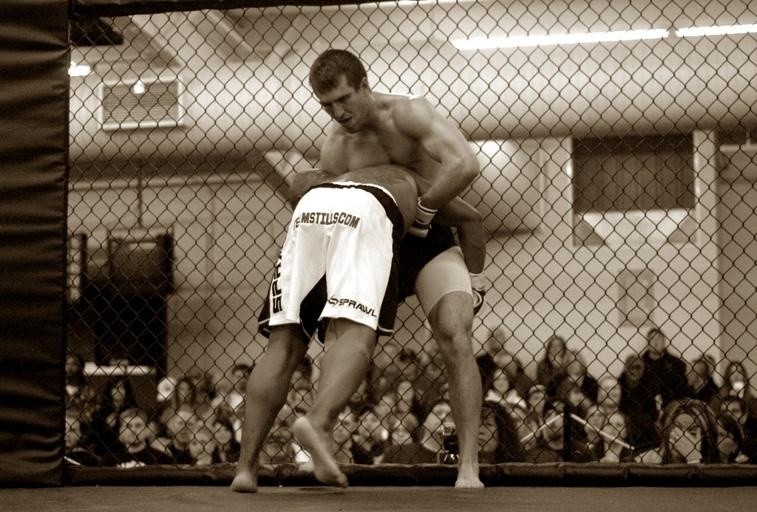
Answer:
[406,197,439,237]
[451,272,485,315]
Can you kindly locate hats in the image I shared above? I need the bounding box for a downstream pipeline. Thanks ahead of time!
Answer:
[156,377,178,402]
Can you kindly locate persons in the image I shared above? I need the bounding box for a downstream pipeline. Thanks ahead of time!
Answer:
[229,162,486,495]
[64,353,317,472]
[474,326,757,465]
[310,48,493,492]
[332,347,457,463]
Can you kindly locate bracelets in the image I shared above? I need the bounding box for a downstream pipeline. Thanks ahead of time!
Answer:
[413,196,438,225]
[467,270,486,293]
